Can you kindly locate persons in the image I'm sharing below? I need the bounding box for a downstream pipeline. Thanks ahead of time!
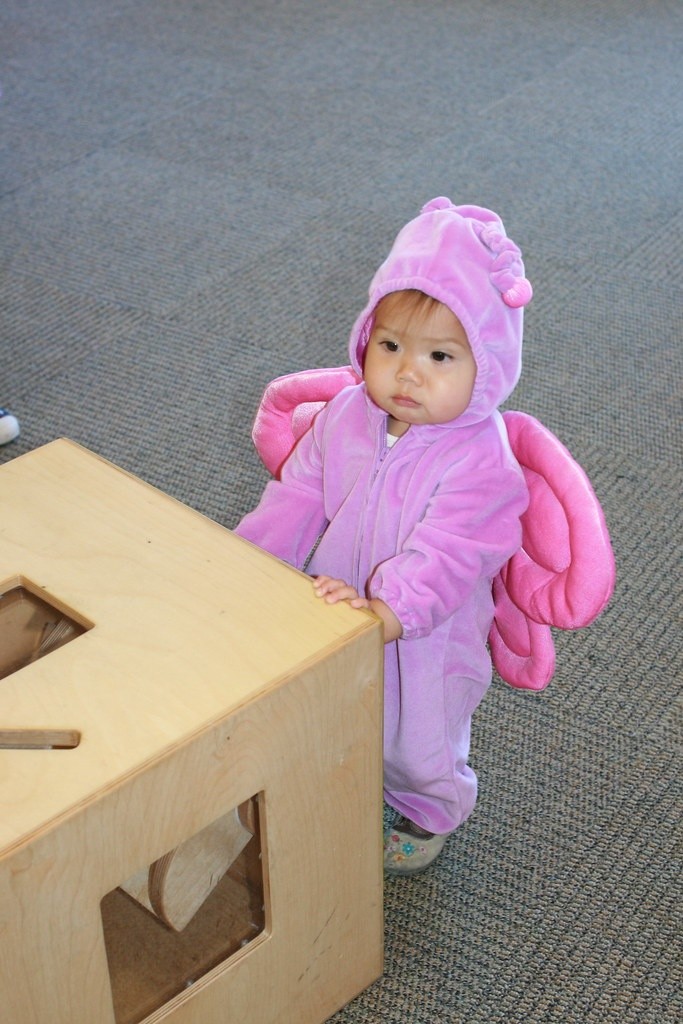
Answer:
[233,194,534,871]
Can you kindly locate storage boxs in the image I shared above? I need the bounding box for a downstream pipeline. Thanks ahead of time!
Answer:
[0,435,384,1024]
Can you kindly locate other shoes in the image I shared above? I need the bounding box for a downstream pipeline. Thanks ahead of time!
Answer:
[384,817,449,874]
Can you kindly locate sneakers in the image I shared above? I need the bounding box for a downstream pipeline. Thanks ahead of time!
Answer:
[0,408,23,446]
